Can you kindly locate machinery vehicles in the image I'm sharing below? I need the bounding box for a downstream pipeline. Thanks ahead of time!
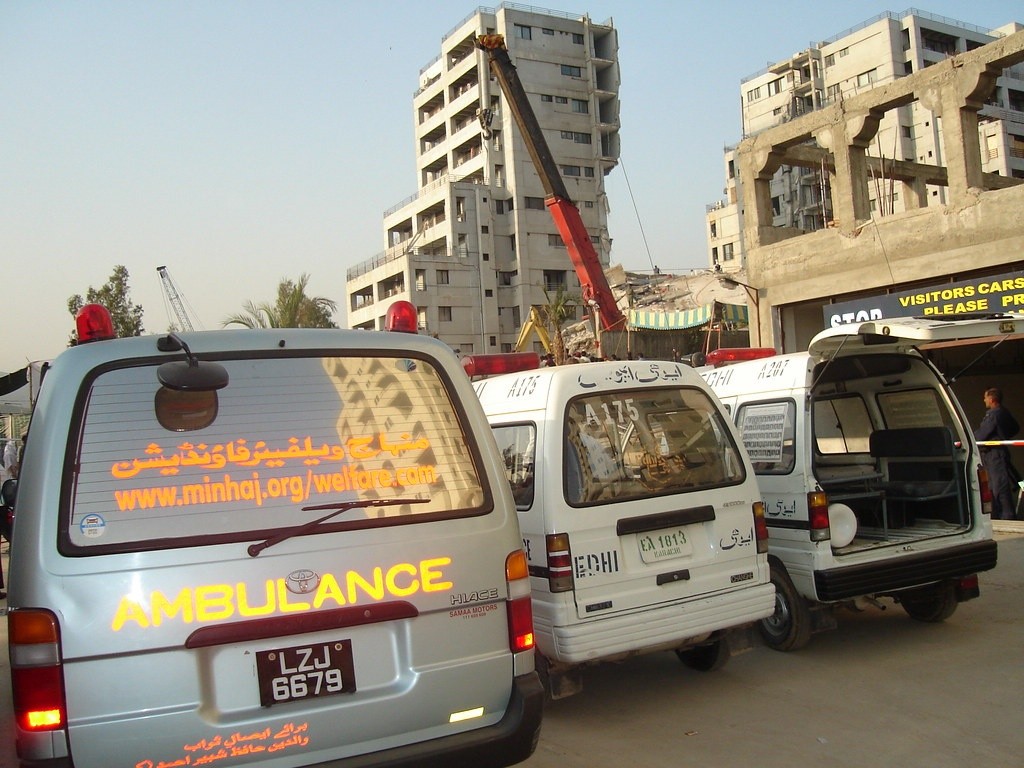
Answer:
[471,30,676,365]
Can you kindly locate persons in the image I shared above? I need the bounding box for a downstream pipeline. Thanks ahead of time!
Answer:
[973,388,1019,520]
[539,348,644,369]
[0,435,27,600]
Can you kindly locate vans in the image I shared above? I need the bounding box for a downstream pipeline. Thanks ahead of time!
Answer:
[0,302,550,768]
[456,350,777,702]
[661,314,1024,654]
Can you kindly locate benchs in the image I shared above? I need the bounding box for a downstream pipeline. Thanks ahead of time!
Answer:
[816,464,874,492]
[861,426,958,502]
